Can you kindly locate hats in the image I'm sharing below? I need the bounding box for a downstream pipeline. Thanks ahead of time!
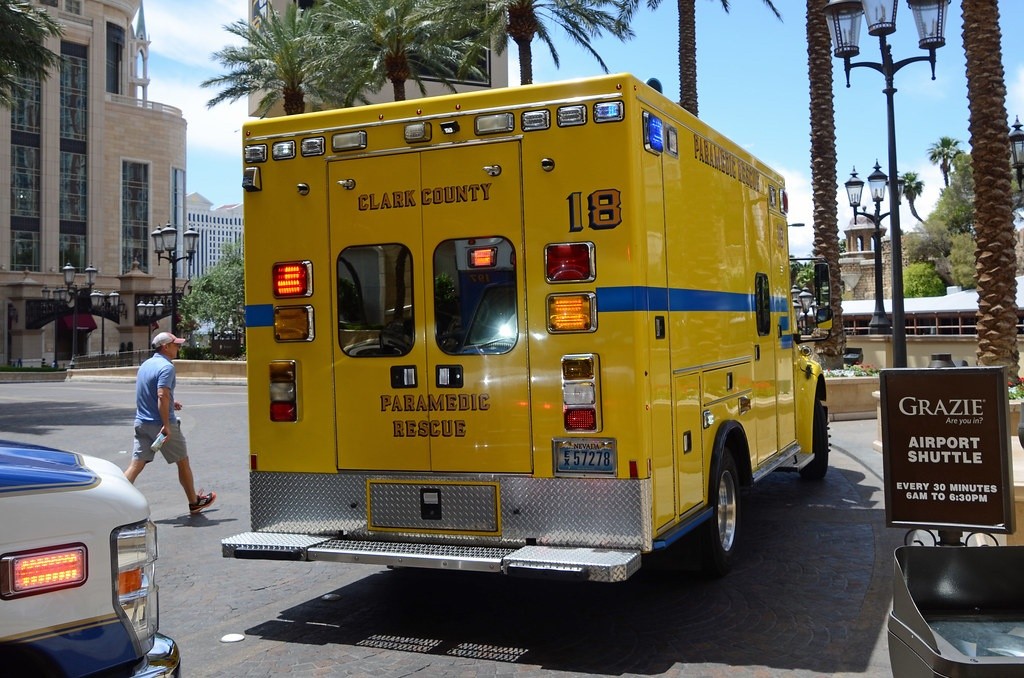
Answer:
[151,331,186,349]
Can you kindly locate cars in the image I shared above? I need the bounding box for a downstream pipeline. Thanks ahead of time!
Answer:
[0,439,182,678]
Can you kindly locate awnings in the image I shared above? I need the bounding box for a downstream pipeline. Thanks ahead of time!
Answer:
[57,313,97,334]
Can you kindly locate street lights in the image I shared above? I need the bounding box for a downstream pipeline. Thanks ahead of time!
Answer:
[843,158,905,334]
[39,284,68,368]
[822,0,952,368]
[1008,114,1024,190]
[150,220,200,335]
[62,262,98,368]
[89,290,119,355]
[137,301,165,349]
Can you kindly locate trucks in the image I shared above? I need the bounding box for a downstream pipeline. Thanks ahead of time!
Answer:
[220,72,833,584]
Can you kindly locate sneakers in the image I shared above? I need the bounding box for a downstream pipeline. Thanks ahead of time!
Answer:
[189,487,216,514]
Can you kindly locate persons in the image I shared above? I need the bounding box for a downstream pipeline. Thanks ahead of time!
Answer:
[51,359,59,369]
[123,331,216,514]
[41,358,47,367]
